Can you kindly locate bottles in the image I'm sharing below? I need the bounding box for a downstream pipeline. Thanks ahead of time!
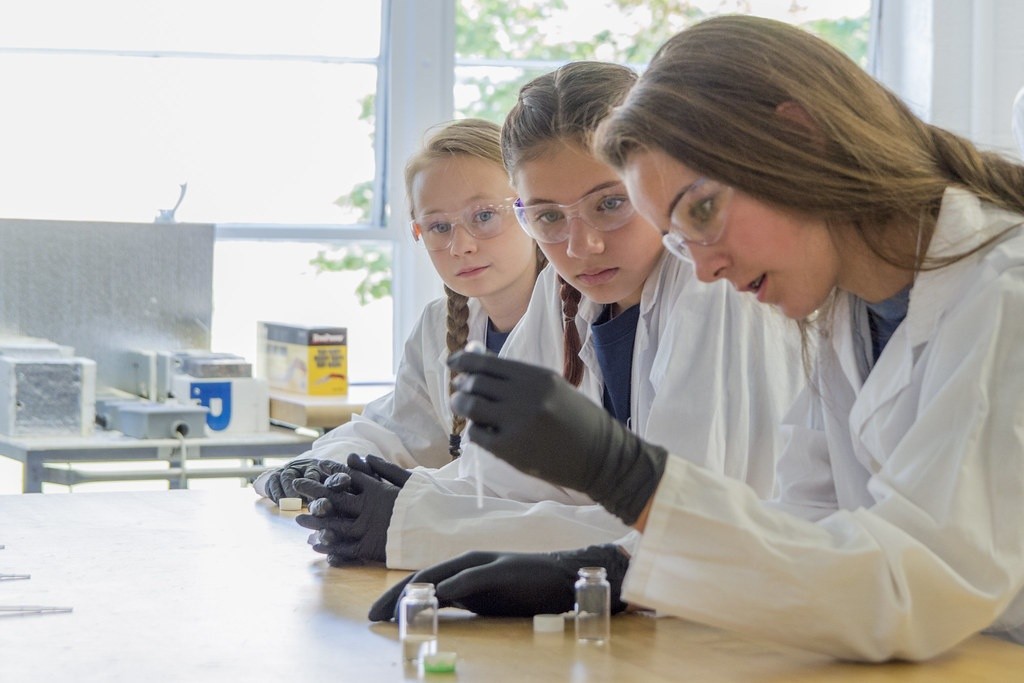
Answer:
[399,583,437,663]
[575,566,610,645]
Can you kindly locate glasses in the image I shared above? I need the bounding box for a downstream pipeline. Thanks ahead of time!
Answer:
[410,195,517,253]
[662,175,733,265]
[512,183,637,243]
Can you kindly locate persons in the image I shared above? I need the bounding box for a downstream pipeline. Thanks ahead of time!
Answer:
[248,118,554,509]
[293,60,818,570]
[366,14,1024,667]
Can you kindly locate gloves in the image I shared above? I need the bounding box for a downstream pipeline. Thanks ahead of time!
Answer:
[263,457,330,508]
[369,541,632,622]
[445,348,668,528]
[291,452,413,569]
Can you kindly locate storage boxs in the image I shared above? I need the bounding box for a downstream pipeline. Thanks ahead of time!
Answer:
[257,321,348,399]
[172,368,271,431]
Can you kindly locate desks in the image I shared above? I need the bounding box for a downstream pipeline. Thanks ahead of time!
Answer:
[0,487,1024,683]
[0,424,318,494]
